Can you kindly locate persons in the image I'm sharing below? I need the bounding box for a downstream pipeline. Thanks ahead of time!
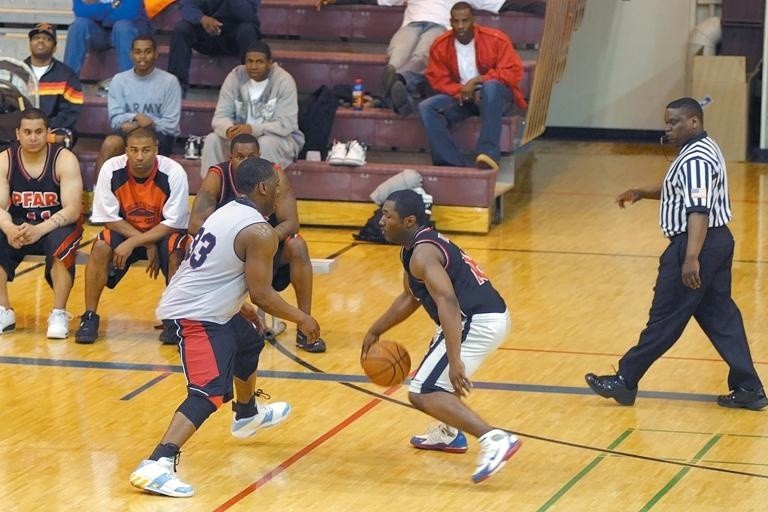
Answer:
[361,190,521,484]
[418,2,528,170]
[22,23,83,149]
[586,98,768,410]
[200,42,303,181]
[64,0,151,98]
[74,127,194,343]
[129,159,320,497]
[92,35,182,190]
[0,109,83,339]
[188,135,325,354]
[315,0,547,119]
[168,0,261,98]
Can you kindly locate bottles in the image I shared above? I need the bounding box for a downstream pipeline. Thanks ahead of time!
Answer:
[354,79,364,110]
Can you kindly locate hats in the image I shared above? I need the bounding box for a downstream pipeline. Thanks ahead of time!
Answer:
[29,23,57,44]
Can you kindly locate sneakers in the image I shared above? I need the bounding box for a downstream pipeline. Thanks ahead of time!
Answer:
[231,401,290,438]
[0,306,17,334]
[46,308,73,339]
[585,371,638,407]
[200,134,208,158]
[717,386,768,410]
[326,140,347,166]
[471,429,522,483]
[75,310,100,344]
[184,136,200,160]
[158,324,178,345]
[344,140,368,166]
[477,153,498,169]
[128,454,193,498]
[294,327,329,352]
[383,64,400,98]
[391,81,411,114]
[410,419,468,453]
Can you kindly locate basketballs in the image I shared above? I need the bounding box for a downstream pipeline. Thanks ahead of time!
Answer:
[363,340,411,386]
